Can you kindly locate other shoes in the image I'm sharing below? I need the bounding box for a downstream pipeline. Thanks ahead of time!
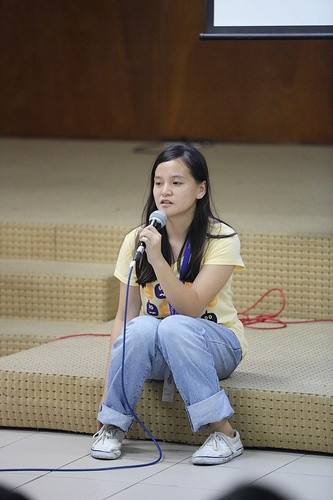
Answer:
[90,424,124,460]
[192,429,245,466]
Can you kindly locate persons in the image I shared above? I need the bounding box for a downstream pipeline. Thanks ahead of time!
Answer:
[89,145,248,466]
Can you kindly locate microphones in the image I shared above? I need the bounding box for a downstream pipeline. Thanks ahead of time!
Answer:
[133,209,167,262]
